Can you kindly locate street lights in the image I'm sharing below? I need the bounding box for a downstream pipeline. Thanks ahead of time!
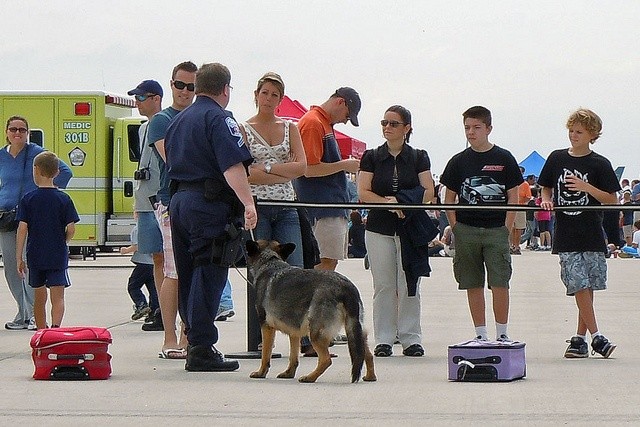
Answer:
[295,199,321,270]
[0,196,20,233]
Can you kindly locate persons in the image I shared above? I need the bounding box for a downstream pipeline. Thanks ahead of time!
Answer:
[518,188,538,250]
[237,72,339,358]
[619,192,637,246]
[510,165,531,255]
[632,180,639,191]
[440,181,460,226]
[15,151,75,329]
[524,175,535,191]
[357,105,433,357]
[165,62,259,372]
[296,87,361,345]
[631,183,640,206]
[147,61,201,359]
[440,104,518,339]
[537,109,619,357]
[126,79,165,329]
[533,188,553,251]
[621,219,640,257]
[347,210,367,258]
[619,179,632,199]
[120,209,162,322]
[0,115,73,330]
[442,223,459,256]
[430,220,441,256]
[344,177,357,220]
[434,176,448,238]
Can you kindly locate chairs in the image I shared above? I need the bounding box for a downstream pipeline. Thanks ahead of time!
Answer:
[403,344,424,356]
[526,245,533,248]
[143,308,162,331]
[564,336,589,358]
[535,247,544,251]
[509,247,514,254]
[591,335,616,358]
[513,248,521,254]
[545,248,551,251]
[530,246,538,250]
[214,309,235,321]
[5,320,29,329]
[331,334,348,344]
[132,305,151,320]
[374,344,393,356]
[28,320,37,330]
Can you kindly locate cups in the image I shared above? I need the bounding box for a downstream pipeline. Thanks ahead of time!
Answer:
[345,111,352,121]
[8,127,28,133]
[134,94,156,102]
[172,80,194,91]
[381,120,404,127]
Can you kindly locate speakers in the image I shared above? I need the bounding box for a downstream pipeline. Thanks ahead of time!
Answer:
[185,343,239,372]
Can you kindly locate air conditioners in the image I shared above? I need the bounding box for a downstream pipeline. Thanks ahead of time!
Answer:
[246,239,376,384]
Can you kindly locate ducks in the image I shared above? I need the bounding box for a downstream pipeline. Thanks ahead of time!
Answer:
[30,328,112,380]
[448,339,526,381]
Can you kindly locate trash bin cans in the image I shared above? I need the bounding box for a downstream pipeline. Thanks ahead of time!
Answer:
[265,163,271,174]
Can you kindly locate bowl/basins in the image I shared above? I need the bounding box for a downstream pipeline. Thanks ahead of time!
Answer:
[389,148,402,156]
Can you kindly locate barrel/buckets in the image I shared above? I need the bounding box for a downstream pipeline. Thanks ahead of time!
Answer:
[159,348,186,359]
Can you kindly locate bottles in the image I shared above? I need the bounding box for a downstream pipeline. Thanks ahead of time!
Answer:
[258,72,284,86]
[337,87,361,127]
[128,80,163,97]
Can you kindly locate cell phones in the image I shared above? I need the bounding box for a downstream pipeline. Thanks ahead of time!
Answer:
[134,169,150,180]
